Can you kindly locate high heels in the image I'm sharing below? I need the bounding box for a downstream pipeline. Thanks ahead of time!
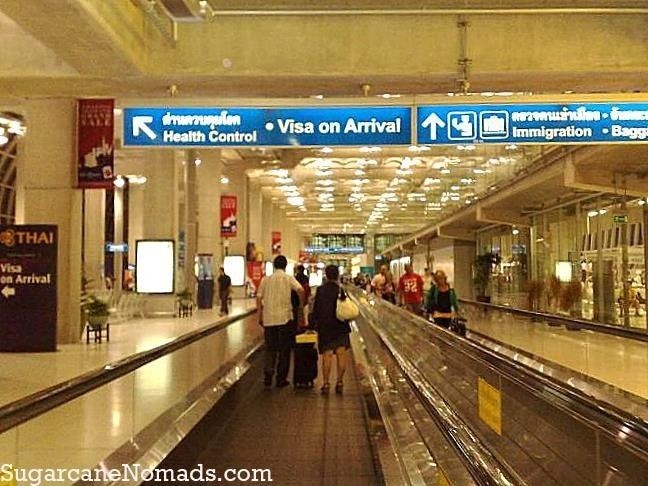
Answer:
[321,383,329,393]
[335,381,344,393]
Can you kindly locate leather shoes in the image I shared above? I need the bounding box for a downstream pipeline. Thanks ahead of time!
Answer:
[262,379,290,386]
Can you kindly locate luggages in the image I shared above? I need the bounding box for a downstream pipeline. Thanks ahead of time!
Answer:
[449,317,468,337]
[293,346,318,387]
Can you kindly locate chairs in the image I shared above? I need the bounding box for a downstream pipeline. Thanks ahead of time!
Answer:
[108,293,149,324]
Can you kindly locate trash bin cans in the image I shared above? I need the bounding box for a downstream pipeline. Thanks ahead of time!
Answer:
[197,274,214,309]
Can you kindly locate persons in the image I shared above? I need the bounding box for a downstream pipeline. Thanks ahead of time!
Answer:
[216,265,232,316]
[354,261,435,315]
[257,254,306,388]
[424,269,460,329]
[309,264,351,396]
[619,280,642,317]
[294,264,311,306]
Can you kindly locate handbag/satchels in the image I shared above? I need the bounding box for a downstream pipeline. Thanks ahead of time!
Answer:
[336,296,359,322]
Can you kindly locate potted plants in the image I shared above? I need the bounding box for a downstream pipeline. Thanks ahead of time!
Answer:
[544,275,561,326]
[560,279,584,330]
[472,253,503,303]
[80,292,115,327]
[523,280,544,322]
